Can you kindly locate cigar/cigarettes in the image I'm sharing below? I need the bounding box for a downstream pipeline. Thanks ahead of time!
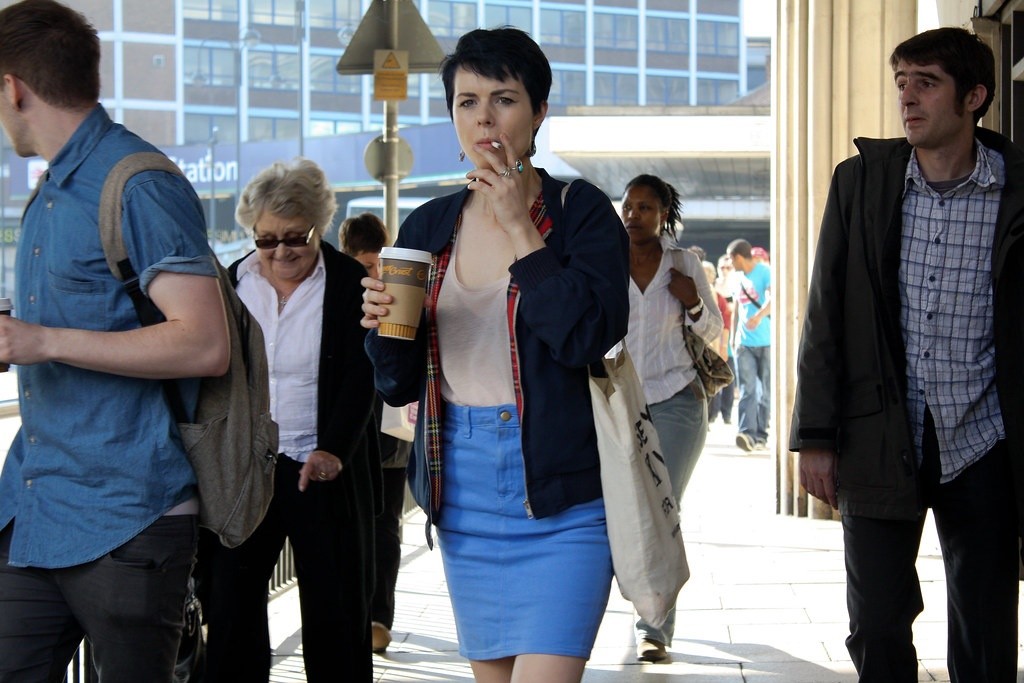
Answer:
[491,141,502,149]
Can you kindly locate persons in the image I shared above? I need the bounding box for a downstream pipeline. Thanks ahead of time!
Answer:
[702,239,770,452]
[604,174,722,659]
[687,246,706,261]
[338,212,410,653]
[360,24,630,682]
[1,1,230,683]
[789,25,1024,682]
[193,159,379,682]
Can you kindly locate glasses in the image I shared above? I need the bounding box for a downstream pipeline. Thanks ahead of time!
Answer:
[250,223,314,249]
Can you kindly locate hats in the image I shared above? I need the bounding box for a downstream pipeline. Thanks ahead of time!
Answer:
[752,247,768,260]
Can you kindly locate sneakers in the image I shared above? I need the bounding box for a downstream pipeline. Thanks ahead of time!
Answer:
[637,637,667,660]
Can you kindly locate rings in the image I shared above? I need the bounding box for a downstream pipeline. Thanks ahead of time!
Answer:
[499,166,513,179]
[511,159,524,172]
[319,471,328,481]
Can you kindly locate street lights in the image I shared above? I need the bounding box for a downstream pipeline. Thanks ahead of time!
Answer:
[191,31,262,240]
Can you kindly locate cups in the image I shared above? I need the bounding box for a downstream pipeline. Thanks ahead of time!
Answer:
[0,298,10,373]
[375,246,434,339]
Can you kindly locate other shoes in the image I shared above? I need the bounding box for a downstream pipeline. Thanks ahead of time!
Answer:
[736,432,765,451]
[370,621,393,651]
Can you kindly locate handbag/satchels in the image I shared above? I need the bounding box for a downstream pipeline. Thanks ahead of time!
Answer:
[98,151,284,549]
[685,325,733,395]
[587,331,690,628]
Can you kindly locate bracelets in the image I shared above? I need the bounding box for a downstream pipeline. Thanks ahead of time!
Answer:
[688,296,703,314]
